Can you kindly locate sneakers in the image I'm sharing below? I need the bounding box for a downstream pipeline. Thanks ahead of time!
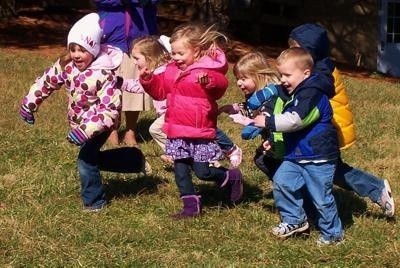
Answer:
[377,180,394,218]
[140,150,153,177]
[108,133,121,148]
[269,221,309,238]
[123,130,140,148]
[82,200,108,212]
[315,231,345,247]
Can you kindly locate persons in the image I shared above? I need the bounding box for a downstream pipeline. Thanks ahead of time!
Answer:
[96,0,159,149]
[130,36,243,169]
[19,12,152,213]
[288,23,396,218]
[218,52,282,182]
[252,46,345,248]
[139,23,244,219]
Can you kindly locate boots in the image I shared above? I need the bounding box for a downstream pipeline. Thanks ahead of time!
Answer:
[172,195,202,221]
[227,144,242,166]
[161,155,174,164]
[221,168,243,201]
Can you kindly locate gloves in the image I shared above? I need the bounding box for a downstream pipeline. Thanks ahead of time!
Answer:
[246,83,278,110]
[218,103,239,115]
[241,121,264,140]
[20,104,35,124]
[67,126,89,146]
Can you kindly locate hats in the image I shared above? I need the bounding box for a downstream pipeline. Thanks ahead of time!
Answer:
[68,13,103,59]
